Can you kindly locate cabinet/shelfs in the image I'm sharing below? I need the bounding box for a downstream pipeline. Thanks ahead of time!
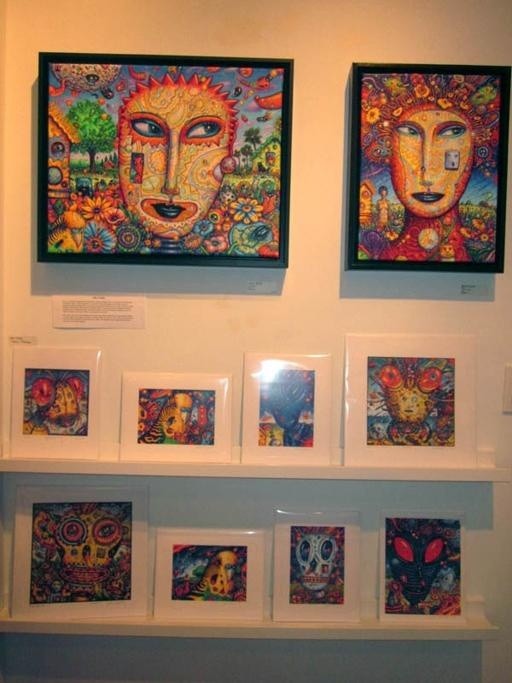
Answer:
[0,456,512,642]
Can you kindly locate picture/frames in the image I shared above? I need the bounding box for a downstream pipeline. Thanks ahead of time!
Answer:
[344,61,512,274]
[37,52,296,269]
